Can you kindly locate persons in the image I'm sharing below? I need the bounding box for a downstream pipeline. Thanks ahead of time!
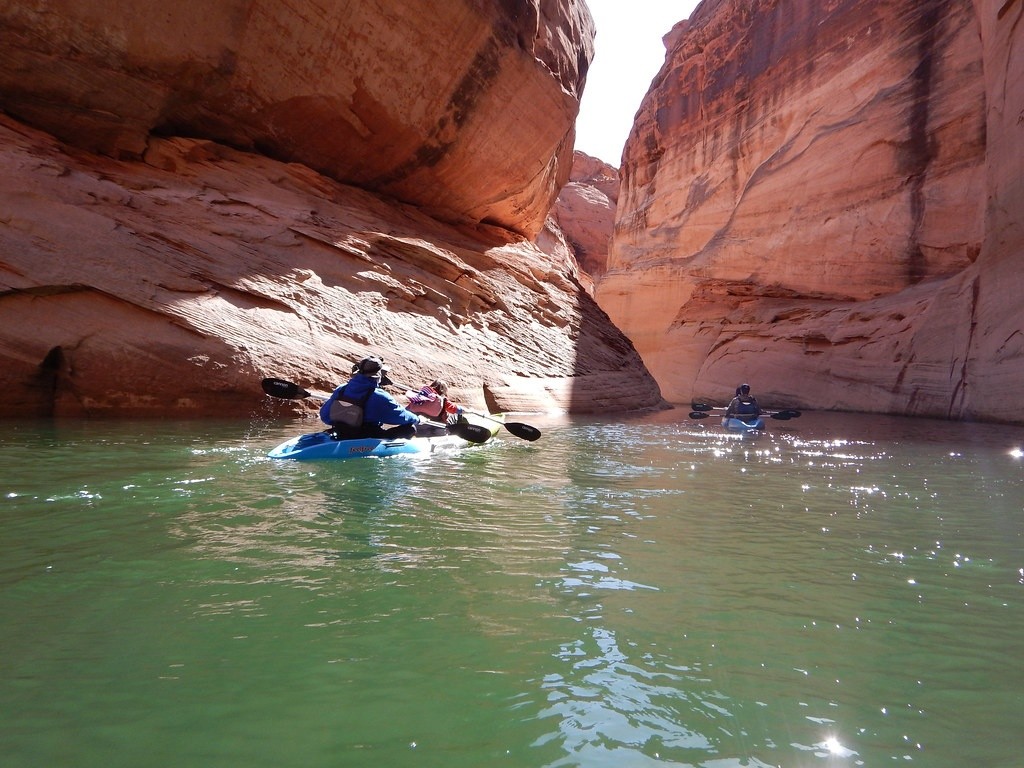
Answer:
[735,387,743,397]
[318,354,431,442]
[725,384,764,424]
[403,377,476,424]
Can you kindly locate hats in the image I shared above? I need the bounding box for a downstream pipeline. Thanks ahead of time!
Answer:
[740,384,750,391]
[430,380,448,397]
[358,355,383,373]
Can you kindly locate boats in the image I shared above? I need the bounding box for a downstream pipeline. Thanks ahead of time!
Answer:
[268,409,509,461]
[720,414,766,434]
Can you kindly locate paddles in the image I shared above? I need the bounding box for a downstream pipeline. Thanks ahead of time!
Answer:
[692,403,801,418]
[261,377,491,443]
[689,412,791,420]
[378,375,542,442]
